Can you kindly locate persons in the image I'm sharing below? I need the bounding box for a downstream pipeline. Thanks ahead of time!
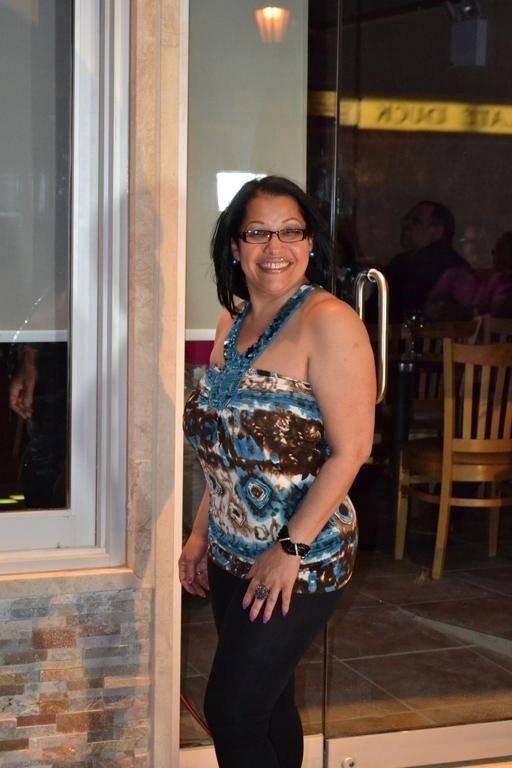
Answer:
[365,200,512,324]
[0,342,67,508]
[183,176,377,768]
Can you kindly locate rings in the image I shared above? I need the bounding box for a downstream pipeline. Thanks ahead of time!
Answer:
[195,571,203,575]
[255,585,270,600]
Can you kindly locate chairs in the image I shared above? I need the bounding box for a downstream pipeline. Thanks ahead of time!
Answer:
[393,311,511,577]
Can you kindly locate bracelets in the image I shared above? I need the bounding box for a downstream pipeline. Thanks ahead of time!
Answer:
[278,524,311,557]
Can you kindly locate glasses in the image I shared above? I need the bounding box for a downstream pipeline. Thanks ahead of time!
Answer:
[235,227,310,245]
